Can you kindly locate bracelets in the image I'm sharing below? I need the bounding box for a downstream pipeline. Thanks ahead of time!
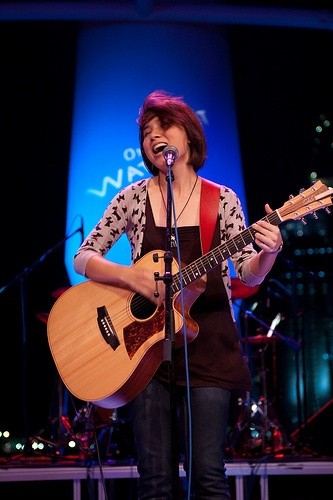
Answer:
[263,240,284,255]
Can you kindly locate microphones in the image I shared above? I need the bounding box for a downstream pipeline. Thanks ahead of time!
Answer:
[162,145,179,166]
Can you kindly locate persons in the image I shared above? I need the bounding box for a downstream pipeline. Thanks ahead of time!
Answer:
[72,90,284,500]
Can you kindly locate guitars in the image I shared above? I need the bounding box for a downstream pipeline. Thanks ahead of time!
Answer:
[46,180,333,409]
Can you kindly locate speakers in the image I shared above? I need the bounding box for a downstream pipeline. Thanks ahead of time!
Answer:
[291,398,333,458]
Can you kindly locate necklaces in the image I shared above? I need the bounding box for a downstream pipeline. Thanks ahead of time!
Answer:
[158,173,199,249]
